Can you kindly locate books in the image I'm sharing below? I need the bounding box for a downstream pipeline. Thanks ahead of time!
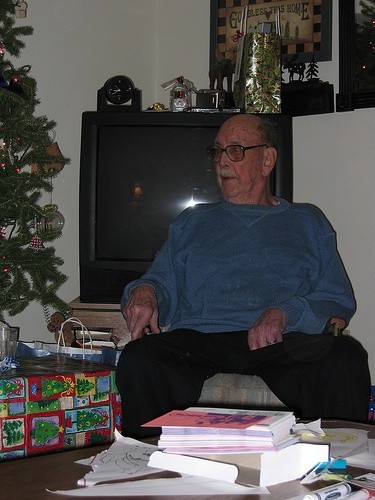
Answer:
[141,406,299,451]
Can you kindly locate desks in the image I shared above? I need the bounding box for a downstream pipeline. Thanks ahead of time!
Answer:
[0,421,375,500]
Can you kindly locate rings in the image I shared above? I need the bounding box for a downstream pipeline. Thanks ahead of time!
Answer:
[267,340,276,344]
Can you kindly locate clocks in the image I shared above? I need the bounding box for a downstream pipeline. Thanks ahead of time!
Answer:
[97,74,142,112]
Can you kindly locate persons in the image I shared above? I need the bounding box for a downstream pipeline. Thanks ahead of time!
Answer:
[111,115,372,439]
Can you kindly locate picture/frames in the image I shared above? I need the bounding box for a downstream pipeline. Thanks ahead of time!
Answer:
[209,0,332,73]
[336,0,375,112]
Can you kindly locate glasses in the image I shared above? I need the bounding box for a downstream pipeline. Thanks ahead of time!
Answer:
[206,144,268,163]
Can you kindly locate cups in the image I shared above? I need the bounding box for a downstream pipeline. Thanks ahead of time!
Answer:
[34,342,43,357]
[4,328,18,359]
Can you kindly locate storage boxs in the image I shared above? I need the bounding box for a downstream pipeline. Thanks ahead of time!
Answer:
[0,355,123,461]
[147,443,329,490]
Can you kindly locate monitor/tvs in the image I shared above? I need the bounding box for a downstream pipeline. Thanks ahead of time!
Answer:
[79,111,293,304]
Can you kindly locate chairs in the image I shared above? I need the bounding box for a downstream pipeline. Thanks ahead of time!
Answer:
[138,316,345,411]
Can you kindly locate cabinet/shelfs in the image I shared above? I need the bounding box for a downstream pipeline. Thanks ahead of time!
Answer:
[47,297,127,347]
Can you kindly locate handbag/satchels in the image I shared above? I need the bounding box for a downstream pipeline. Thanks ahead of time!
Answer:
[16,317,117,367]
